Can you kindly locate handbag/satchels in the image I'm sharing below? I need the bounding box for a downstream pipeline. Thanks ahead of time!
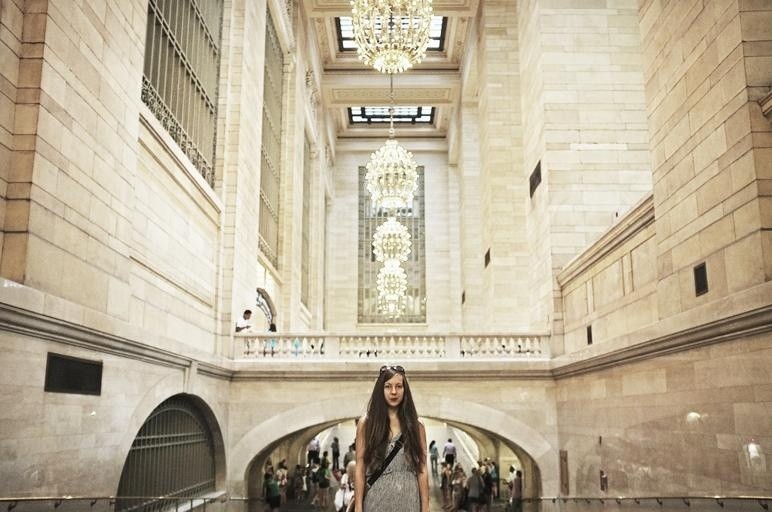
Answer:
[334,488,344,511]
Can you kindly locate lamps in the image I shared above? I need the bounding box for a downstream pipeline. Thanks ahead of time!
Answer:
[351,1,433,323]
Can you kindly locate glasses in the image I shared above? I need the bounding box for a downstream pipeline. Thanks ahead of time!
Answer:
[380,366,404,372]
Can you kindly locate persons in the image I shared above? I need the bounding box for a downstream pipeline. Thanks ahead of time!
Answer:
[263,323,277,358]
[353,364,430,512]
[261,435,358,512]
[236,309,253,356]
[439,456,522,512]
[442,438,457,469]
[428,440,440,478]
